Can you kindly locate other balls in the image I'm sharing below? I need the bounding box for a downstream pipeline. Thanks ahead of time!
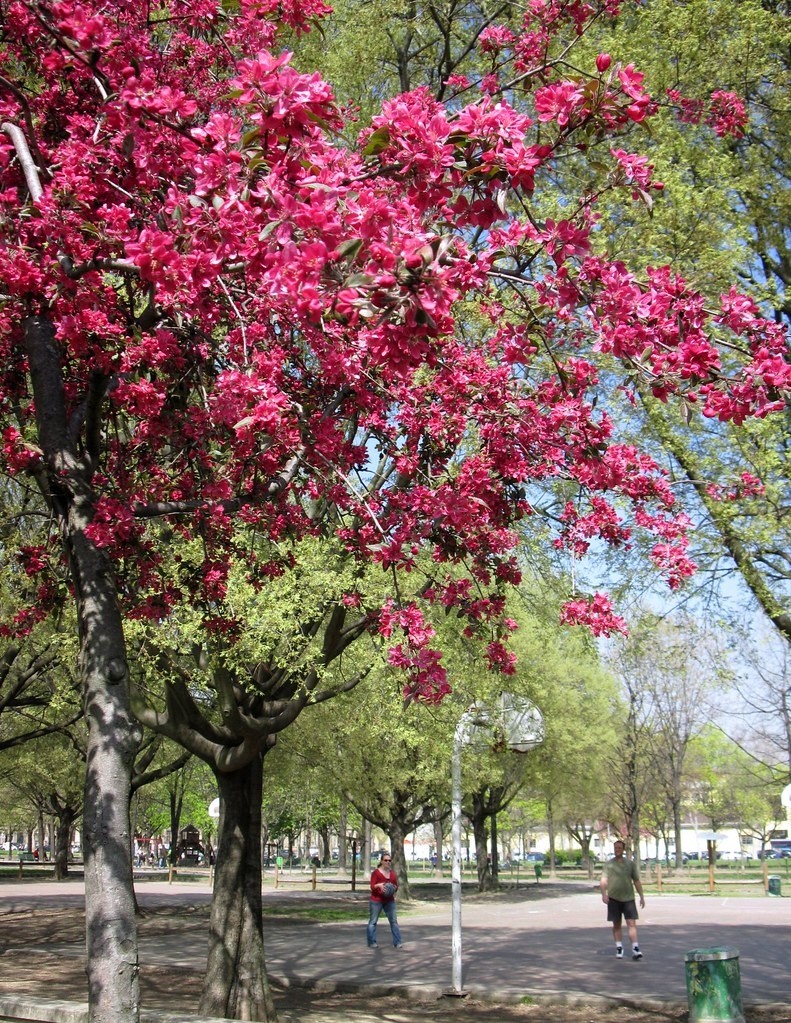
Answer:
[383,883,394,897]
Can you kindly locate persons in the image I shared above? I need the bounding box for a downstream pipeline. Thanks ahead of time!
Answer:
[364,851,404,951]
[598,839,647,960]
[0,843,443,868]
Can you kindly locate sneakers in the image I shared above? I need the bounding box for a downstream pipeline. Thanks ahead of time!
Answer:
[616,946,624,959]
[393,944,402,948]
[632,946,642,959]
[368,943,378,948]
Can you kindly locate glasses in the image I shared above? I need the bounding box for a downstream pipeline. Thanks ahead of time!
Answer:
[383,859,391,862]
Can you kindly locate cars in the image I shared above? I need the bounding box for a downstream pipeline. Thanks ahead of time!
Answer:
[527,847,791,863]
[263,848,391,864]
[0,840,83,853]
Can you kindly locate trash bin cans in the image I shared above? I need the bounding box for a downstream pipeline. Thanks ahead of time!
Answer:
[767,874,781,897]
[683,946,745,1023]
